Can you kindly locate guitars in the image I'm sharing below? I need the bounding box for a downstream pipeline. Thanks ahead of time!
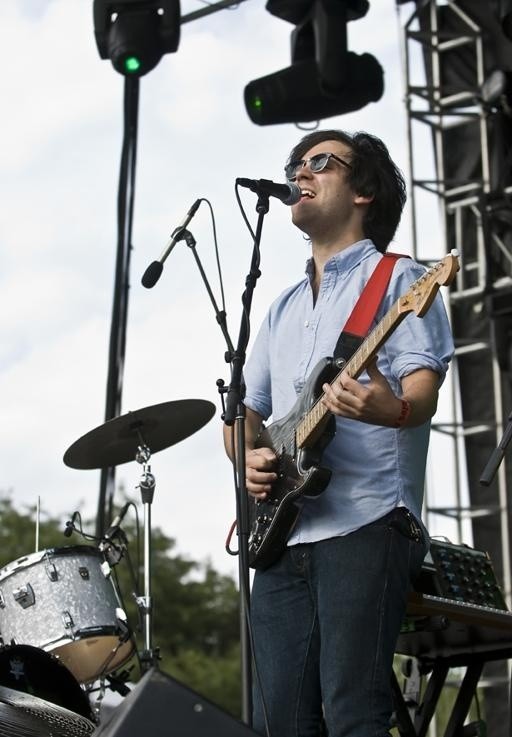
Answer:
[248,255,460,568]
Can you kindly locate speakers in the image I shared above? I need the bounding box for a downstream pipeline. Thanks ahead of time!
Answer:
[91,665,264,737]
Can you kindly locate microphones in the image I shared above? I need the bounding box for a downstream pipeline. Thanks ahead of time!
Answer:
[236,178,301,205]
[142,198,201,288]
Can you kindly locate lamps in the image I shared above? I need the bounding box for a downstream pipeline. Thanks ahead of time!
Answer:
[92,0,386,125]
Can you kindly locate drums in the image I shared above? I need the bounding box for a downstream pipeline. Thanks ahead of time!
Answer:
[0,545,135,683]
[0,645,101,737]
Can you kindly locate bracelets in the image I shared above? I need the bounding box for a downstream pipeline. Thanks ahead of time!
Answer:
[391,399,413,428]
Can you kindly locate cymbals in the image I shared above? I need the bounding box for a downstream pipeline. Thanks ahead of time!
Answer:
[63,401,215,470]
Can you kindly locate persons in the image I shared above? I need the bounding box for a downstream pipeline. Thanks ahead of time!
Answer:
[223,130,455,737]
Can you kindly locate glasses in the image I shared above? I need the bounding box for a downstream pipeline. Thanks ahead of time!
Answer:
[283,151,353,180]
